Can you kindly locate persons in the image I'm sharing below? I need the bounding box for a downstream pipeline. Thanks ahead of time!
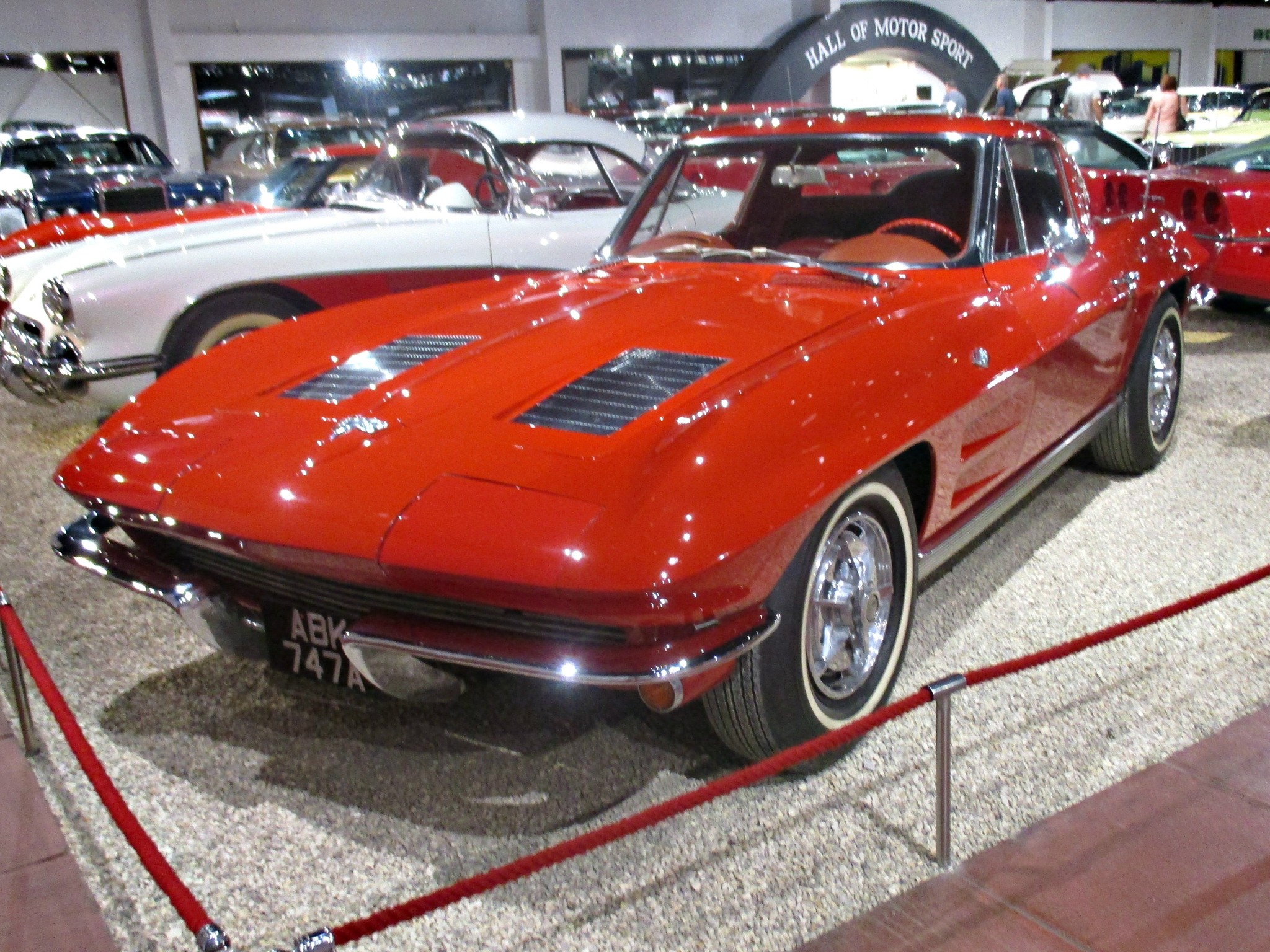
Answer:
[1142,75,1187,140]
[1063,63,1102,160]
[995,74,1017,117]
[944,80,966,110]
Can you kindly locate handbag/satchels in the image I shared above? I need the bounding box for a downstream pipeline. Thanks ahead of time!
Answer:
[1176,110,1187,131]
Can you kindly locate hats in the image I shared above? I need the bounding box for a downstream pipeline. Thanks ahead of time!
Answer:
[1076,64,1090,75]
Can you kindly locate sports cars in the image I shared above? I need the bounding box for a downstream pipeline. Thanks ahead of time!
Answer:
[43,101,1210,771]
[0,63,1270,374]
